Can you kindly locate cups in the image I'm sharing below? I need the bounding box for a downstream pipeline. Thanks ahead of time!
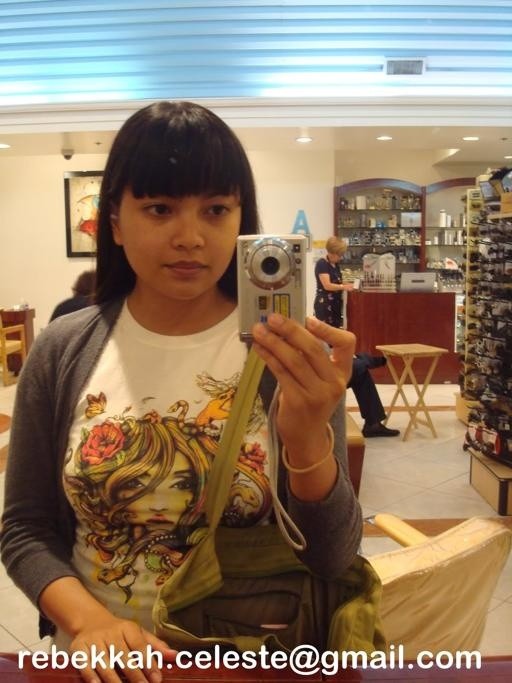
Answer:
[440,209,446,227]
[434,237,438,244]
[446,216,451,227]
[353,279,360,289]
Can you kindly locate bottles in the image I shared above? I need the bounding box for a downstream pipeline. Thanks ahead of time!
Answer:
[361,214,366,227]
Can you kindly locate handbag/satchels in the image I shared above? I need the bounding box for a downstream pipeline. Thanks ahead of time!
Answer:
[151,526,386,664]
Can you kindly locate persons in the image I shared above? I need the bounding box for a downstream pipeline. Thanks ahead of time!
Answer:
[314,236,355,347]
[50,270,101,322]
[347,352,401,436]
[1,101,363,681]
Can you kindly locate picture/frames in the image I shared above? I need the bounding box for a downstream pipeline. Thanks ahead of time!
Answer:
[64,171,104,257]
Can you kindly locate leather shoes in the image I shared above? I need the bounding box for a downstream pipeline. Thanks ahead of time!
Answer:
[362,421,399,437]
[355,354,386,367]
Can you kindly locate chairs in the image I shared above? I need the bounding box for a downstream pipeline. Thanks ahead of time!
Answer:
[0,319,26,386]
[369,513,512,657]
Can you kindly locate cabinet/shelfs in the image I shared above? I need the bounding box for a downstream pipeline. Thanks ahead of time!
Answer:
[334,178,476,353]
[467,189,512,516]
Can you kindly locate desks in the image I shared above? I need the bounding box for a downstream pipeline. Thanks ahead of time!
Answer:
[1,309,35,371]
[376,343,449,442]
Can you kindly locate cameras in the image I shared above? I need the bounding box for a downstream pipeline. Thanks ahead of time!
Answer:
[234,232,308,343]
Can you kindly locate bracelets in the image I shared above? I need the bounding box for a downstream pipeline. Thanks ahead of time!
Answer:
[274,418,337,474]
